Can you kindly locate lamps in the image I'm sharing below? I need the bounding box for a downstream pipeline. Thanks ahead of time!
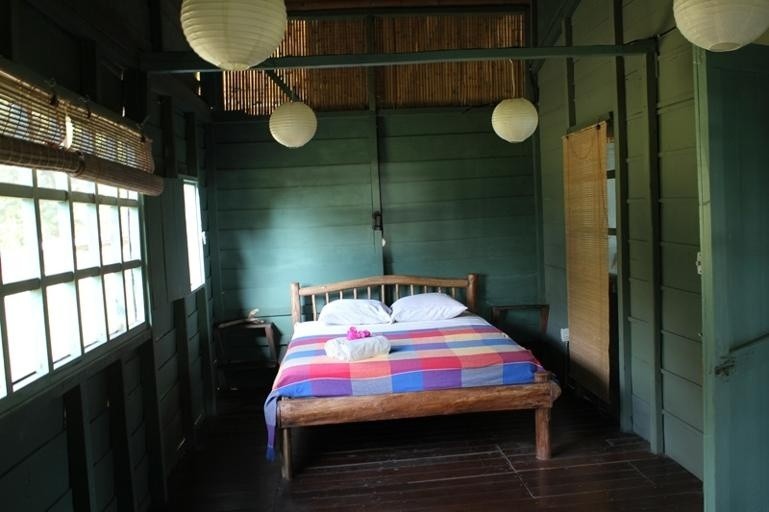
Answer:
[491,60,540,142]
[269,86,317,148]
[179,0,288,71]
[673,0,768,53]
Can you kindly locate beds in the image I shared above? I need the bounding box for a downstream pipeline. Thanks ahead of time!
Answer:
[263,271,563,481]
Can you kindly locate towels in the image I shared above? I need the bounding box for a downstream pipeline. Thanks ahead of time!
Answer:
[323,334,393,361]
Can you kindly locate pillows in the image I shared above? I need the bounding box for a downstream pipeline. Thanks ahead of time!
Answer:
[318,298,392,326]
[390,291,469,322]
